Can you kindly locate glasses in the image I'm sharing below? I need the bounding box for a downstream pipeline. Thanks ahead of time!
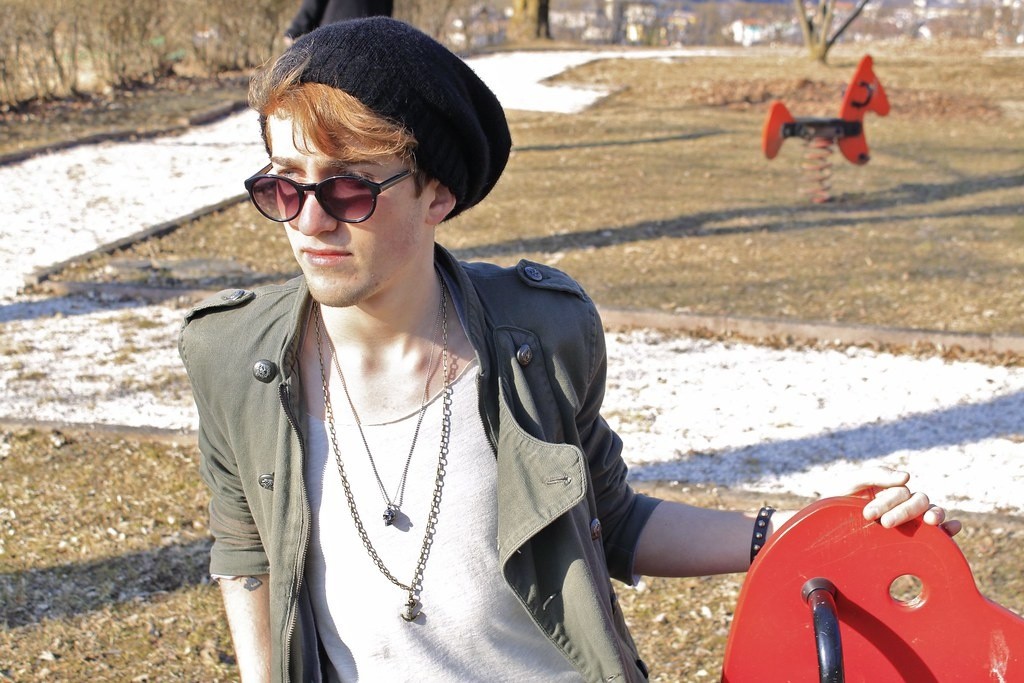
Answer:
[242,161,418,222]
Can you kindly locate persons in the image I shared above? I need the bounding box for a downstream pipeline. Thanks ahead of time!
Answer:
[173,15,968,683]
[280,0,397,47]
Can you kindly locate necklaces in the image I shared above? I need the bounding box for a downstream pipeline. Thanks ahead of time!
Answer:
[315,267,452,624]
[315,264,443,525]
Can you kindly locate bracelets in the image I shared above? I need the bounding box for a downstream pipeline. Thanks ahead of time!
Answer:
[747,505,775,567]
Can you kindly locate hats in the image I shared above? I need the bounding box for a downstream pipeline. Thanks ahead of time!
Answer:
[258,17,513,222]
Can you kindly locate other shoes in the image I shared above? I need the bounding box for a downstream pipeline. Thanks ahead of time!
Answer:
[535,33,555,41]
